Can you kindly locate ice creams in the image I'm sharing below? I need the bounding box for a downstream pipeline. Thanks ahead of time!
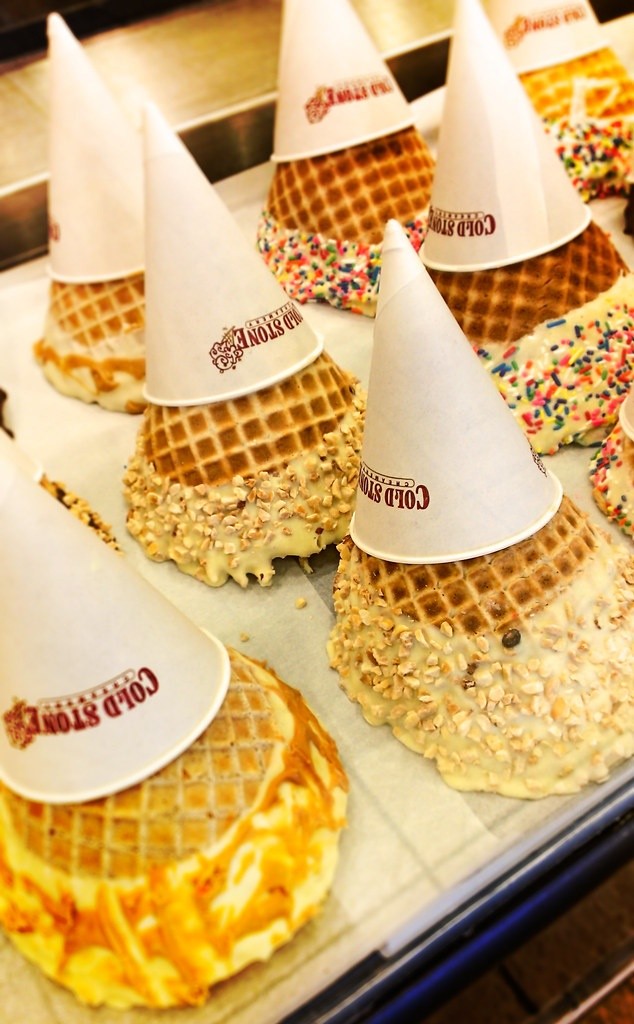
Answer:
[0,41,634,1013]
